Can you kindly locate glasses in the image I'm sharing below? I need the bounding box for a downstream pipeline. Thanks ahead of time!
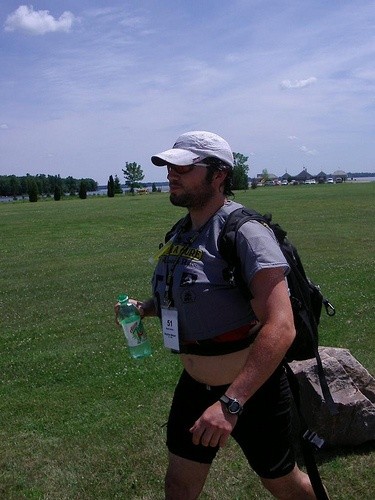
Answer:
[167,164,209,174]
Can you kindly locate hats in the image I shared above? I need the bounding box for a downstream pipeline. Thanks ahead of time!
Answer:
[150,130,235,168]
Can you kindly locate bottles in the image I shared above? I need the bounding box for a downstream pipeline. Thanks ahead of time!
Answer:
[116,292,152,359]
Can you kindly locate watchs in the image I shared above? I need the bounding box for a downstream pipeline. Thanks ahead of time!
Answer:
[219,394,244,417]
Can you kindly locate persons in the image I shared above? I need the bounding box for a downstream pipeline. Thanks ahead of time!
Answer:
[114,130,331,500]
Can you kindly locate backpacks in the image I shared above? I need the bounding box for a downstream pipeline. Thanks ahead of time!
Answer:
[217,207,335,362]
